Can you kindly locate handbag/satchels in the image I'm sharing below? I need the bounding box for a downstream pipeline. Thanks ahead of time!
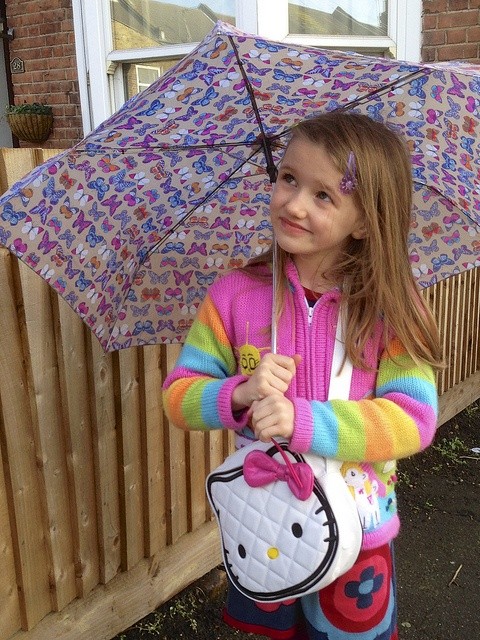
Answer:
[204,435,362,603]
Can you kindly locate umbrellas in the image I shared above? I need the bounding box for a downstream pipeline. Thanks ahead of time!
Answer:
[0,22,480,353]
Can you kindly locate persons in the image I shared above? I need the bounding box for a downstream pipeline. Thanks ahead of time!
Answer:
[161,113,443,639]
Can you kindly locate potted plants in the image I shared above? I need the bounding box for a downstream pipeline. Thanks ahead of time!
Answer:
[1,102,53,144]
